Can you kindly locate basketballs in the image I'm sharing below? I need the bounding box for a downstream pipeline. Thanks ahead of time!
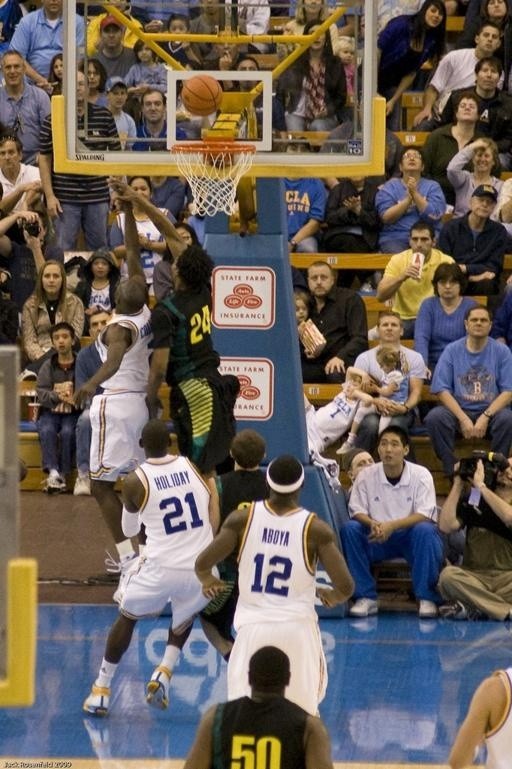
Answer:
[181,76,224,117]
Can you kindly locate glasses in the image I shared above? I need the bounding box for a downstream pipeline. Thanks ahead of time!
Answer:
[403,152,422,159]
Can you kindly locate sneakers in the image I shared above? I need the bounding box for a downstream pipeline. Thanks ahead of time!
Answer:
[81,686,110,717]
[73,477,91,496]
[336,441,355,454]
[349,598,380,617]
[146,668,170,709]
[46,477,67,493]
[418,599,436,618]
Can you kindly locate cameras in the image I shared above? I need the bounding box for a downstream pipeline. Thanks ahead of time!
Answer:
[21,219,40,238]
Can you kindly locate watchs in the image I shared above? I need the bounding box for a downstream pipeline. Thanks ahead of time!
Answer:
[482,410,495,420]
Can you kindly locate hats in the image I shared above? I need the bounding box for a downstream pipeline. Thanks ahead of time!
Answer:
[473,184,498,202]
[100,14,123,32]
[104,75,126,94]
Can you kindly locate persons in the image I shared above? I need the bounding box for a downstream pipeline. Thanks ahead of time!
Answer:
[190,0,235,67]
[75,247,119,317]
[130,88,187,152]
[335,346,408,458]
[22,258,85,364]
[491,279,512,353]
[279,137,328,283]
[354,309,426,459]
[421,91,501,206]
[377,0,447,133]
[145,13,203,73]
[108,176,178,309]
[324,170,382,291]
[177,645,335,769]
[83,416,220,718]
[448,664,512,769]
[86,0,144,58]
[205,30,238,93]
[0,51,50,165]
[151,221,201,300]
[332,35,358,130]
[304,365,376,461]
[0,136,47,237]
[300,259,370,385]
[38,54,63,99]
[438,454,512,622]
[35,320,79,495]
[414,262,480,384]
[447,137,504,224]
[374,145,446,254]
[496,176,512,229]
[90,15,138,79]
[74,309,128,496]
[0,0,28,52]
[293,291,308,326]
[0,210,65,308]
[105,177,235,619]
[280,0,338,58]
[423,305,512,488]
[411,23,505,135]
[375,220,456,340]
[105,75,137,151]
[198,427,271,663]
[226,0,271,54]
[232,57,287,141]
[341,448,375,508]
[457,0,512,66]
[79,58,110,109]
[38,71,122,250]
[7,0,86,97]
[64,174,165,578]
[442,56,512,158]
[340,424,449,619]
[124,39,168,95]
[193,453,355,726]
[436,183,508,304]
[276,18,346,144]
[140,172,185,226]
